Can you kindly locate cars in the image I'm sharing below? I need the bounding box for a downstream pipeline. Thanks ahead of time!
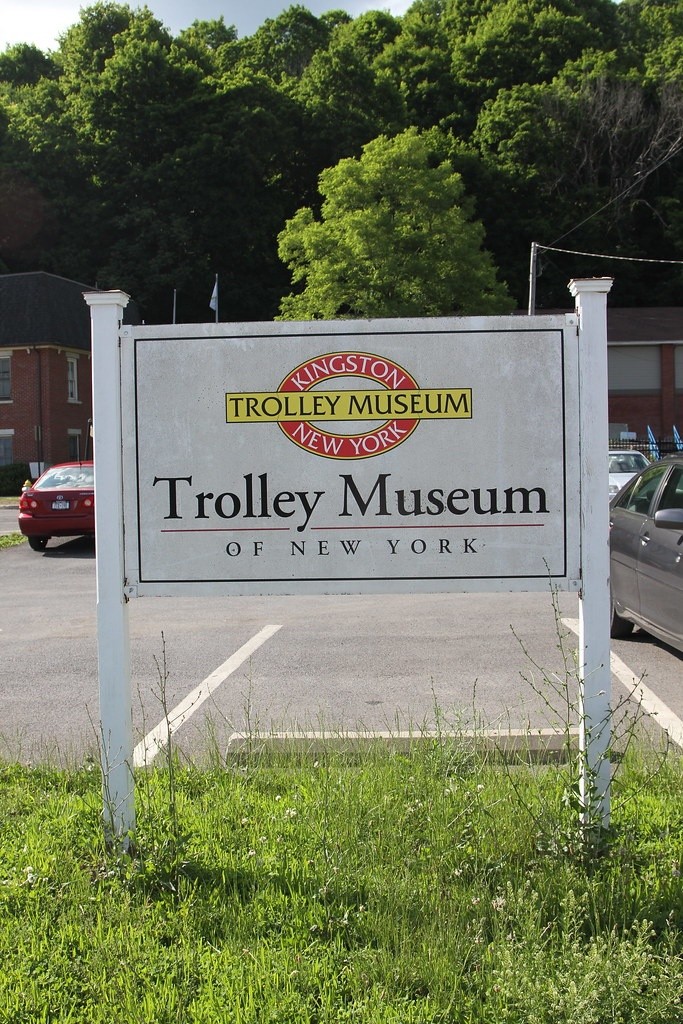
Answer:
[18,460,94,550]
[609,451,651,502]
[608,456,683,653]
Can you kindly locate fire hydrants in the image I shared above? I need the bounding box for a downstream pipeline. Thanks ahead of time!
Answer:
[21,479,33,493]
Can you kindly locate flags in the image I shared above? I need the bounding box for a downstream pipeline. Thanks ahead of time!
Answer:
[673,426,683,449]
[647,426,659,461]
[210,282,217,310]
